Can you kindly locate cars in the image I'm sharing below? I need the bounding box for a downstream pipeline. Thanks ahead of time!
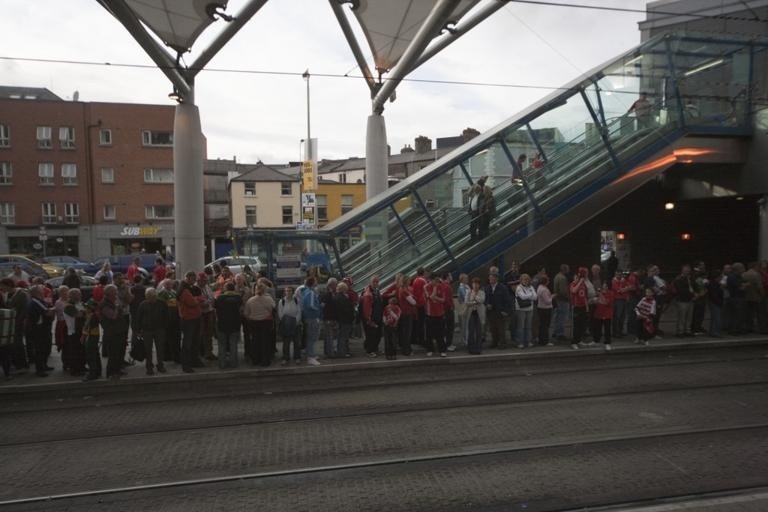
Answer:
[0,251,176,303]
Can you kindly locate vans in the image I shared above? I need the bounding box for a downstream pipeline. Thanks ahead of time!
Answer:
[204,255,265,276]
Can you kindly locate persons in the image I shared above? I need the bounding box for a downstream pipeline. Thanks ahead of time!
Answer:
[127,255,140,283]
[7,260,30,288]
[628,93,652,131]
[94,258,113,282]
[457,260,668,356]
[465,183,497,246]
[153,256,166,288]
[130,261,278,376]
[529,154,549,191]
[0,272,130,381]
[276,267,455,366]
[604,250,617,271]
[512,154,527,191]
[670,259,768,338]
[63,267,80,289]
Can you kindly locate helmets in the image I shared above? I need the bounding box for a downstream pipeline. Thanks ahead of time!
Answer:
[343,277,354,290]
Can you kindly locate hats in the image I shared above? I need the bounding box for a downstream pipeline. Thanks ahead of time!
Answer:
[96,275,111,285]
[196,272,210,281]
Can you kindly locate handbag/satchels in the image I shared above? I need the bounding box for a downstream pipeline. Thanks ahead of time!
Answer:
[130,336,145,362]
[516,296,531,308]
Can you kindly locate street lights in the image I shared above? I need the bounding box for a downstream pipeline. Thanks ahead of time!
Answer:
[301,68,314,162]
[298,139,306,223]
